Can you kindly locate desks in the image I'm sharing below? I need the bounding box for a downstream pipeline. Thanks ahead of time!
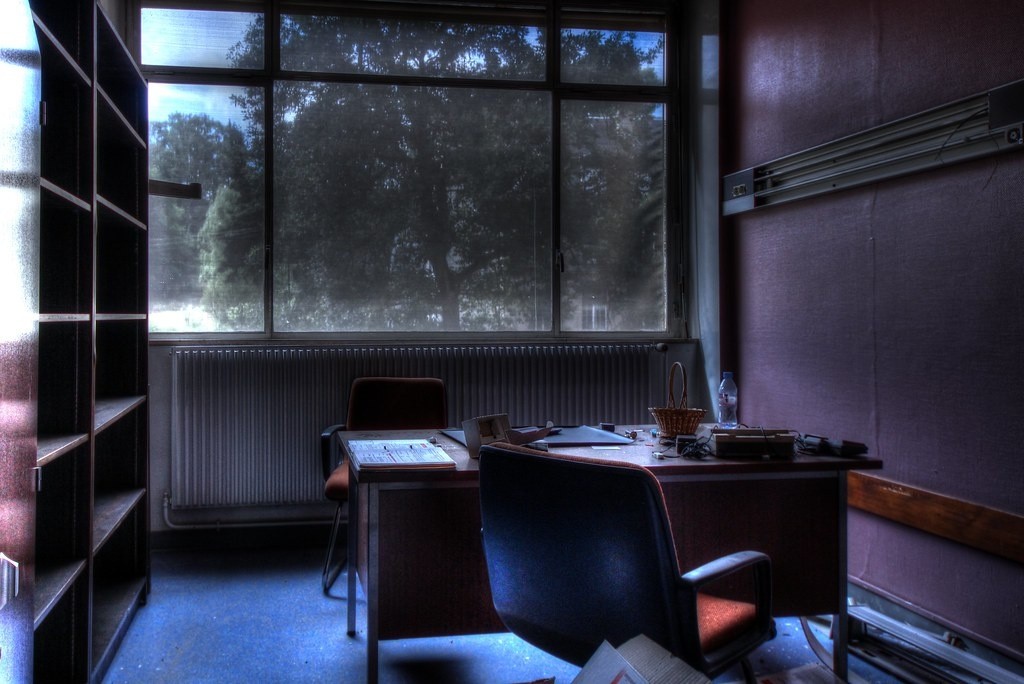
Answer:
[341,430,885,683]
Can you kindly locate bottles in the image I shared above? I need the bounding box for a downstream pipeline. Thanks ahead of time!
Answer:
[718,372,737,429]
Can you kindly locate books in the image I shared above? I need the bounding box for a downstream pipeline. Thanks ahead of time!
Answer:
[347,439,456,472]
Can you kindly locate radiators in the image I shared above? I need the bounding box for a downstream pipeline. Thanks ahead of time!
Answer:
[162,342,674,532]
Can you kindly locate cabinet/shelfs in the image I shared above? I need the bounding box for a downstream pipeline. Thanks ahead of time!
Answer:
[0,0,159,684]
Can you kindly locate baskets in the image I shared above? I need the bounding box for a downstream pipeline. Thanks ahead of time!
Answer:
[647,362,707,437]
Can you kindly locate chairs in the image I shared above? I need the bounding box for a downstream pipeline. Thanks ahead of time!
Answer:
[477,441,780,684]
[322,377,455,592]
